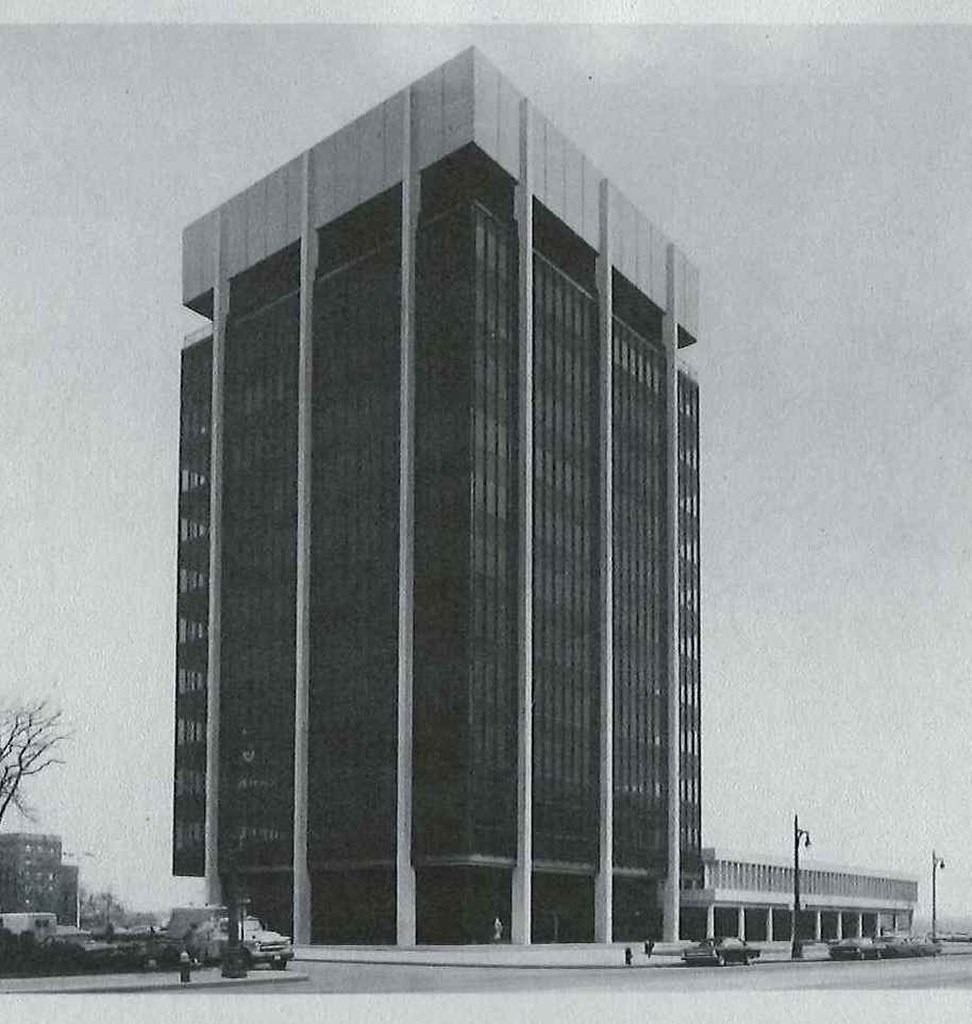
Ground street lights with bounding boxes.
[930,849,945,938]
[790,812,813,961]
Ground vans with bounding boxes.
[1,912,57,947]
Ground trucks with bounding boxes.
[169,904,295,973]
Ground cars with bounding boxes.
[904,935,941,957]
[50,919,177,969]
[868,936,914,959]
[829,936,885,961]
[682,935,760,965]
